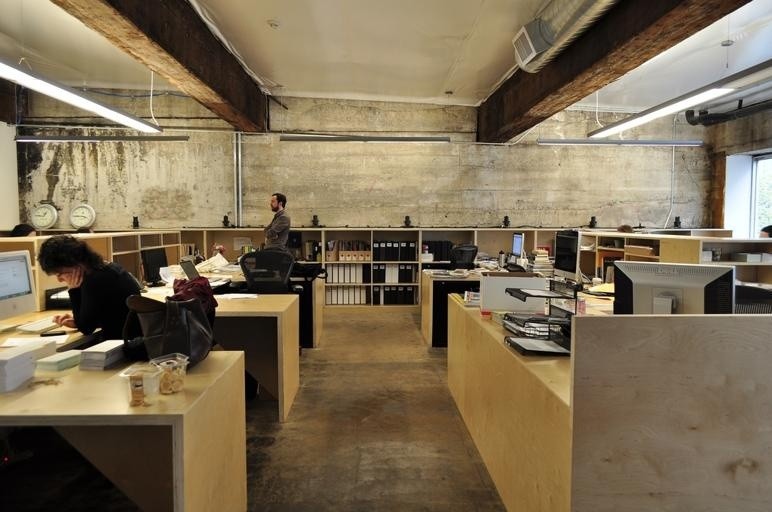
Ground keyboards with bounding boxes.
[16,314,63,334]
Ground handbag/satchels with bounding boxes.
[279,263,328,283]
[122,294,214,370]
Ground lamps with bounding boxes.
[586,13,772,140]
[1,0,163,135]
[278,86,454,144]
[13,56,190,142]
[537,123,704,147]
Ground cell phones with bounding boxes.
[40,330,66,337]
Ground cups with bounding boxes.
[555,276,567,282]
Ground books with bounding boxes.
[422,253,434,262]
[431,268,470,278]
[0,339,127,393]
[527,249,554,272]
[463,290,480,307]
[702,250,772,262]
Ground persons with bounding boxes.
[263,192,293,249]
[36,233,148,362]
[618,224,635,233]
[75,227,96,233]
[759,225,772,238]
[9,223,37,237]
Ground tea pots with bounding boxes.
[592,278,602,287]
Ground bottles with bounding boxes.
[578,298,587,315]
[499,250,504,268]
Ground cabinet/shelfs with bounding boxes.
[32,224,771,306]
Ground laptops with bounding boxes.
[180,260,222,283]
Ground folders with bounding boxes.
[373,286,380,304]
[373,240,416,261]
[373,264,385,283]
[384,286,414,304]
[399,264,412,283]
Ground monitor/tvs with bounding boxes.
[554,230,583,285]
[0,250,38,331]
[140,247,168,287]
[614,260,735,315]
[513,232,526,259]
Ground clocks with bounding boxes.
[69,204,96,229]
[31,204,57,230]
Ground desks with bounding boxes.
[447,293,772,512]
[1,294,300,423]
[1,350,247,512]
[142,272,323,348]
[423,267,551,346]
